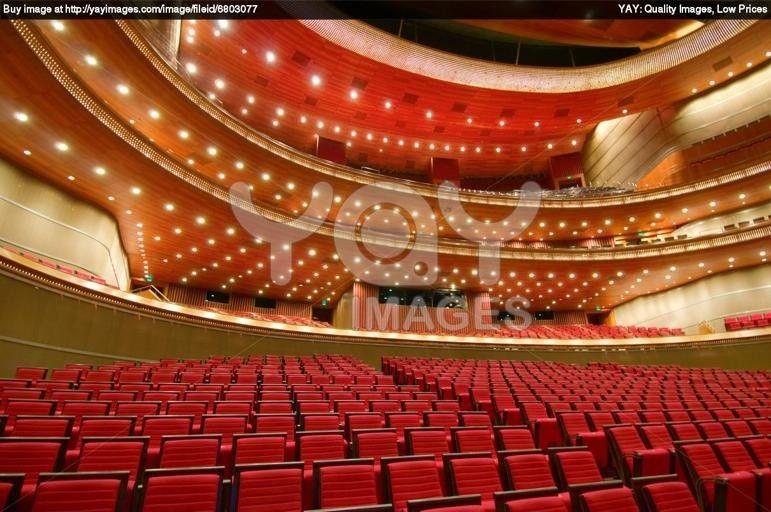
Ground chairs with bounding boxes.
[239,312,771,337]
[0,355,771,512]
